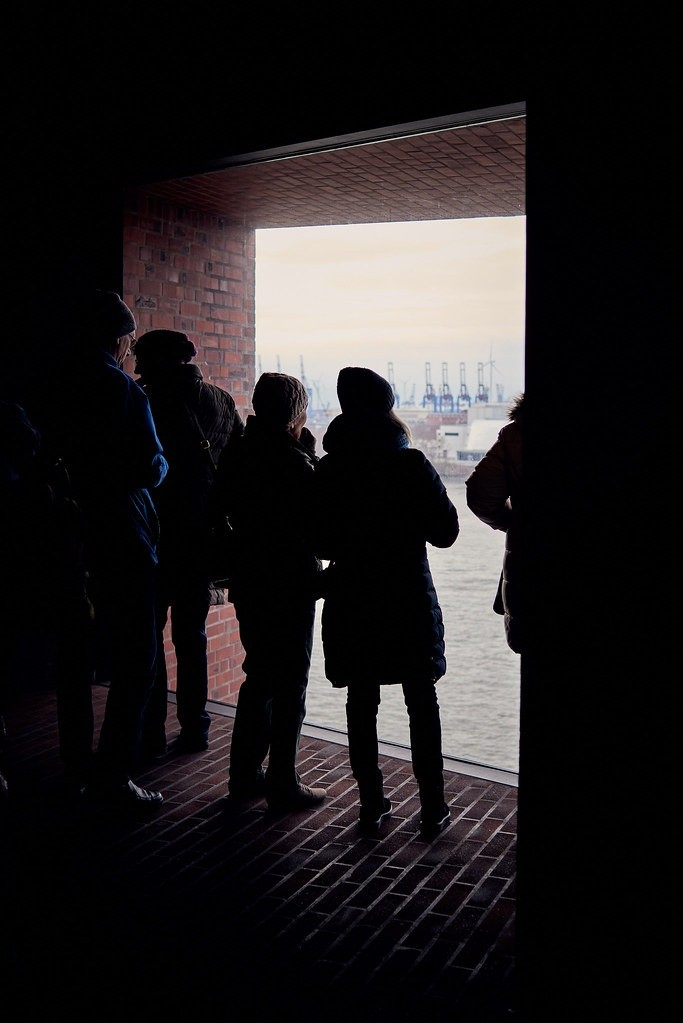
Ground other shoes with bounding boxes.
[359,798,391,823]
[420,803,451,824]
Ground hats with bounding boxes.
[338,369,394,413]
[87,289,136,341]
[253,373,307,424]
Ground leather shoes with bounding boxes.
[84,778,164,812]
[267,786,325,812]
[228,776,267,798]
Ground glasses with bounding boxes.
[128,333,138,347]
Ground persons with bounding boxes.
[303,367,453,832]
[224,372,332,811]
[465,393,524,658]
[0,281,241,815]
